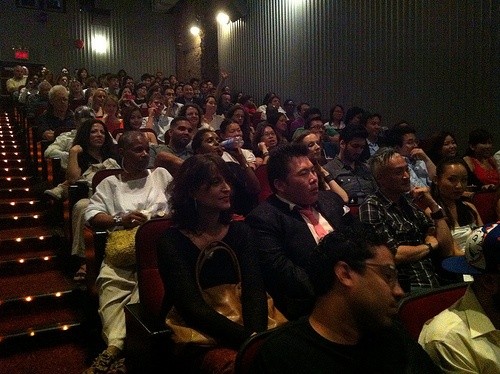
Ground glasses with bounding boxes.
[360,261,397,281]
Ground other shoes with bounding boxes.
[82,349,125,374]
[73,263,87,281]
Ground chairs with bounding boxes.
[14,90,500,374]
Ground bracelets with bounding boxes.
[430,209,444,219]
[424,243,433,250]
[114,215,122,224]
[324,175,334,183]
[263,152,269,157]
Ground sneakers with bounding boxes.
[48,183,65,199]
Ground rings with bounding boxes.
[132,219,135,222]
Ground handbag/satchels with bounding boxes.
[166,240,289,345]
[106,225,138,268]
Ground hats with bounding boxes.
[442,221,500,277]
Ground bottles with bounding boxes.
[218,135,244,153]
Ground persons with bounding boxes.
[7,65,500,374]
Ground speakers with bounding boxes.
[225,0,249,23]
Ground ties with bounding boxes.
[299,206,328,239]
